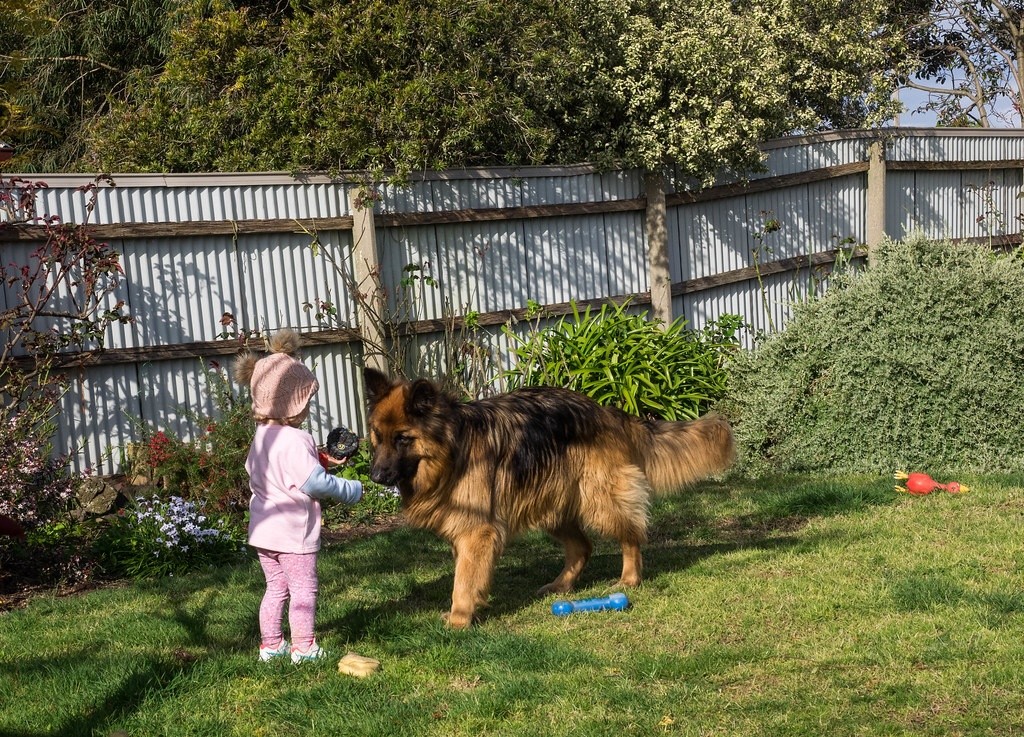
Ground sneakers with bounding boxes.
[291,637,334,665]
[258,633,291,663]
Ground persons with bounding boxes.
[234,328,362,664]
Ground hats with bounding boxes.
[232,327,319,418]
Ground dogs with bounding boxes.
[363,367,735,636]
